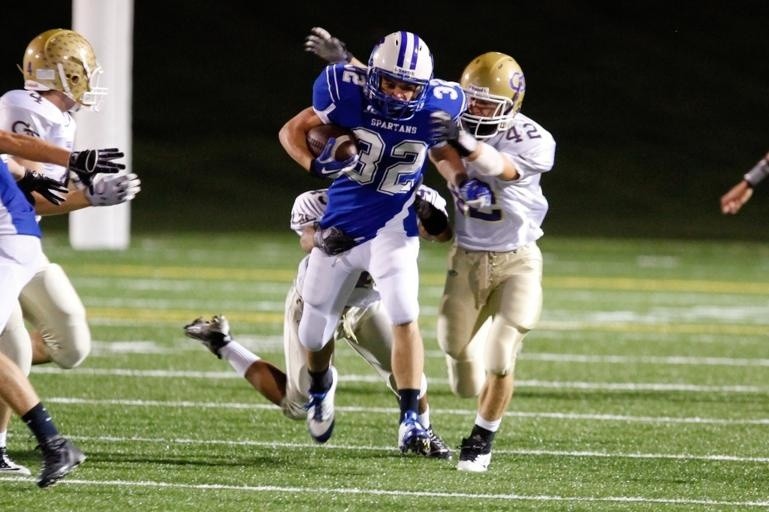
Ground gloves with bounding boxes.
[309,137,359,179]
[313,226,356,255]
[304,27,353,64]
[430,110,477,157]
[16,168,69,207]
[453,177,496,217]
[413,184,447,236]
[85,173,141,206]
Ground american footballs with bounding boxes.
[307,126,358,161]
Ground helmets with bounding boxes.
[23,29,107,113]
[364,31,434,121]
[460,52,526,139]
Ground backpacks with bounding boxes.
[69,148,125,196]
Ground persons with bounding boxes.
[0,26,144,476]
[305,22,557,473]
[0,128,128,489]
[720,148,769,218]
[271,25,496,455]
[182,176,454,459]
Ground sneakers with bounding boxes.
[33,434,88,488]
[425,424,449,459]
[0,446,30,475]
[456,437,491,472]
[398,410,431,456]
[304,365,338,444]
[183,316,231,359]
